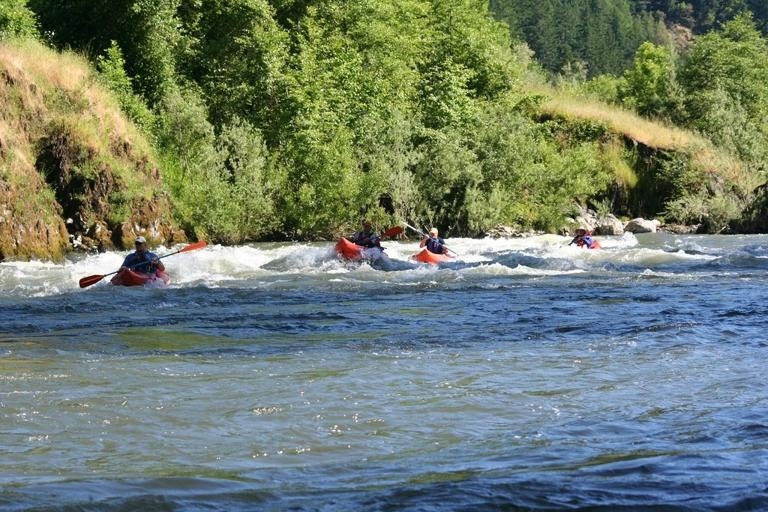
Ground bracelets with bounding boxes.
[158,260,161,264]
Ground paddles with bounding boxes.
[79,240,206,287]
[402,221,458,255]
[369,226,402,240]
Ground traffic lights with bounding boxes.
[576,240,600,249]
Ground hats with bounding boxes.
[575,226,587,232]
[134,236,146,243]
[430,228,438,239]
[363,221,371,228]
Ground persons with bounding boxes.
[119,236,165,278]
[420,228,448,254]
[568,224,593,249]
[352,222,378,248]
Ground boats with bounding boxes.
[334,237,381,260]
[111,266,170,287]
[411,247,449,264]
